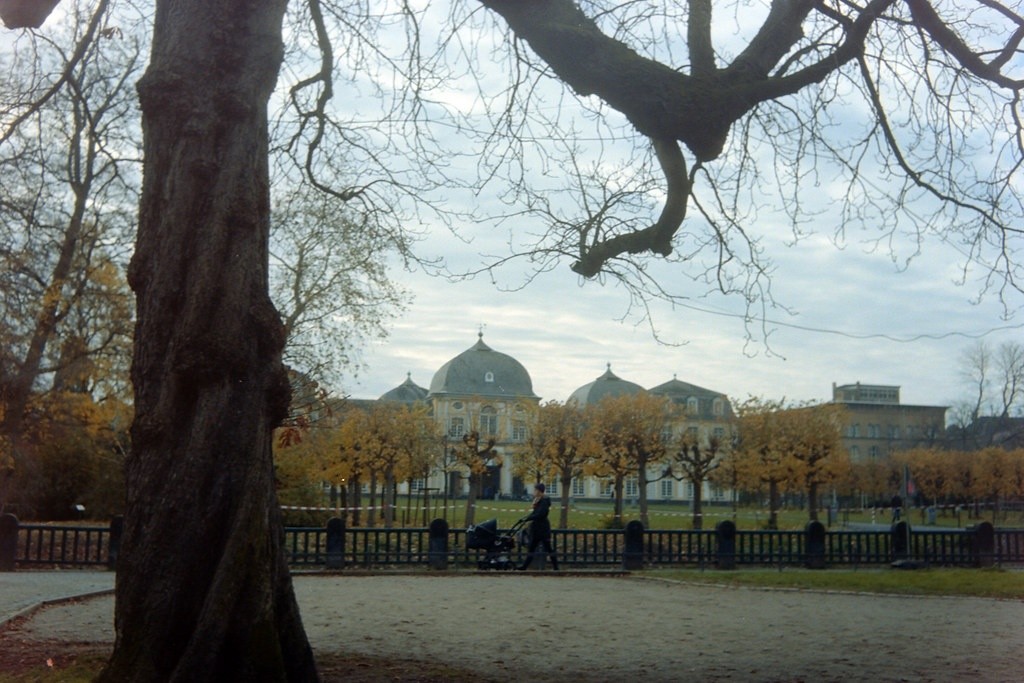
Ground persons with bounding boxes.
[515,483,559,571]
[890,490,903,523]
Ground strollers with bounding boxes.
[466,516,525,571]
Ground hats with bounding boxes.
[535,484,545,493]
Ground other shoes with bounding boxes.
[554,565,559,570]
[516,567,526,570]
